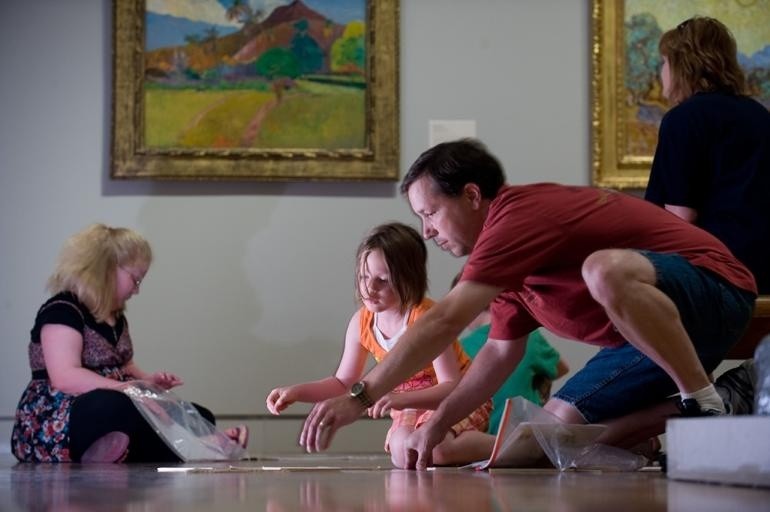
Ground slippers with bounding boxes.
[80,432,129,463]
[218,425,249,455]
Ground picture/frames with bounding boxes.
[588,0,770,192]
[111,0,403,183]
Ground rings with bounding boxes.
[317,420,326,429]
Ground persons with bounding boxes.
[445,267,565,434]
[299,140,759,471]
[10,225,250,464]
[643,15,767,296]
[269,224,497,467]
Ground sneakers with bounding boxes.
[647,358,756,470]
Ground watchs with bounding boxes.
[348,378,374,409]
